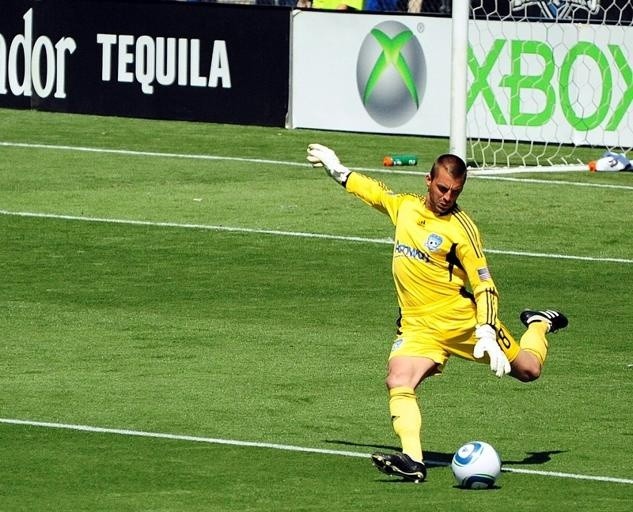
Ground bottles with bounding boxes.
[383,154,418,166]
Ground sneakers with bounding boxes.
[520,310,569,333]
[371,452,427,484]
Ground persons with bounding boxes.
[362,0,452,12]
[296,0,363,13]
[306,144,569,485]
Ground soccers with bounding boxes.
[450,441,500,489]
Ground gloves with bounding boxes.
[306,142,352,188]
[473,325,515,378]
